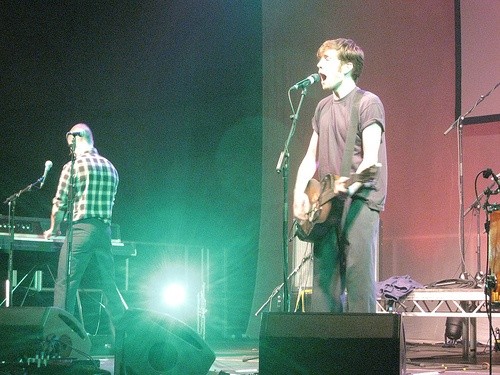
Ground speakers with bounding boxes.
[114,308,216,375]
[258,311,407,375]
[0,306,92,363]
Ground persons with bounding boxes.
[43,123,130,353]
[292,38,388,313]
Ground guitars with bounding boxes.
[291,165,381,243]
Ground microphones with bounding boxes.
[290,73,320,90]
[67,130,84,136]
[40,160,53,188]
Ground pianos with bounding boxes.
[0,215,138,259]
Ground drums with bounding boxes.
[487,201,500,309]
